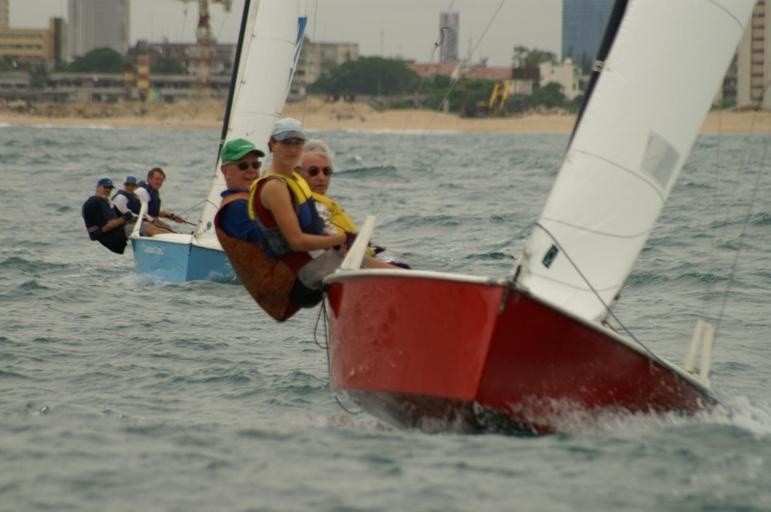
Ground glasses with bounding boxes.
[309,167,333,176]
[239,162,262,170]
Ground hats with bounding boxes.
[124,176,137,185]
[100,179,115,188]
[272,117,305,141]
[221,139,265,163]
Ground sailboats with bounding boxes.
[124,1,328,292]
[318,0,763,442]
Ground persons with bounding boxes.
[294,139,386,257]
[213,139,412,322]
[82,177,169,249]
[137,167,183,228]
[111,174,174,236]
[247,116,404,290]
